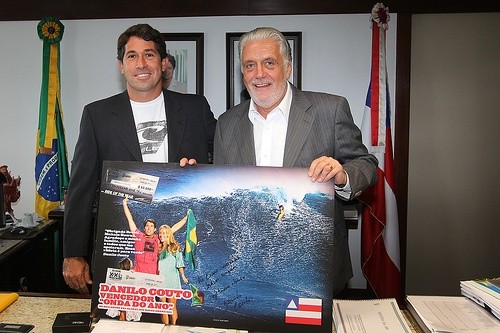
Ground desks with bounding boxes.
[0,203,500,333]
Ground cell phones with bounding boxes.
[0,323,34,333]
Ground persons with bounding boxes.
[117,197,190,325]
[62,23,216,296]
[213,27,379,298]
[162,52,176,89]
[0,165,22,221]
[358,2,403,310]
[35,16,69,221]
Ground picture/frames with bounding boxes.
[160,32,205,96]
[225,31,302,112]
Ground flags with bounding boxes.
[189,283,204,308]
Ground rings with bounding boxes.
[326,163,333,170]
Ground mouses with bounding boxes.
[11,227,28,234]
